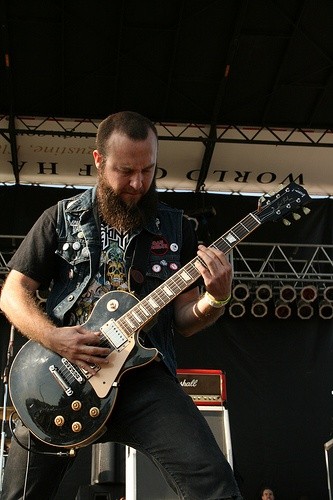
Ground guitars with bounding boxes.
[8,180,314,449]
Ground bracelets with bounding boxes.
[205,291,231,308]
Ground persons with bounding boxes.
[262,488,275,500]
[0,111,244,500]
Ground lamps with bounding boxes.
[230,283,333,320]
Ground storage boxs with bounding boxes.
[125,406,235,500]
